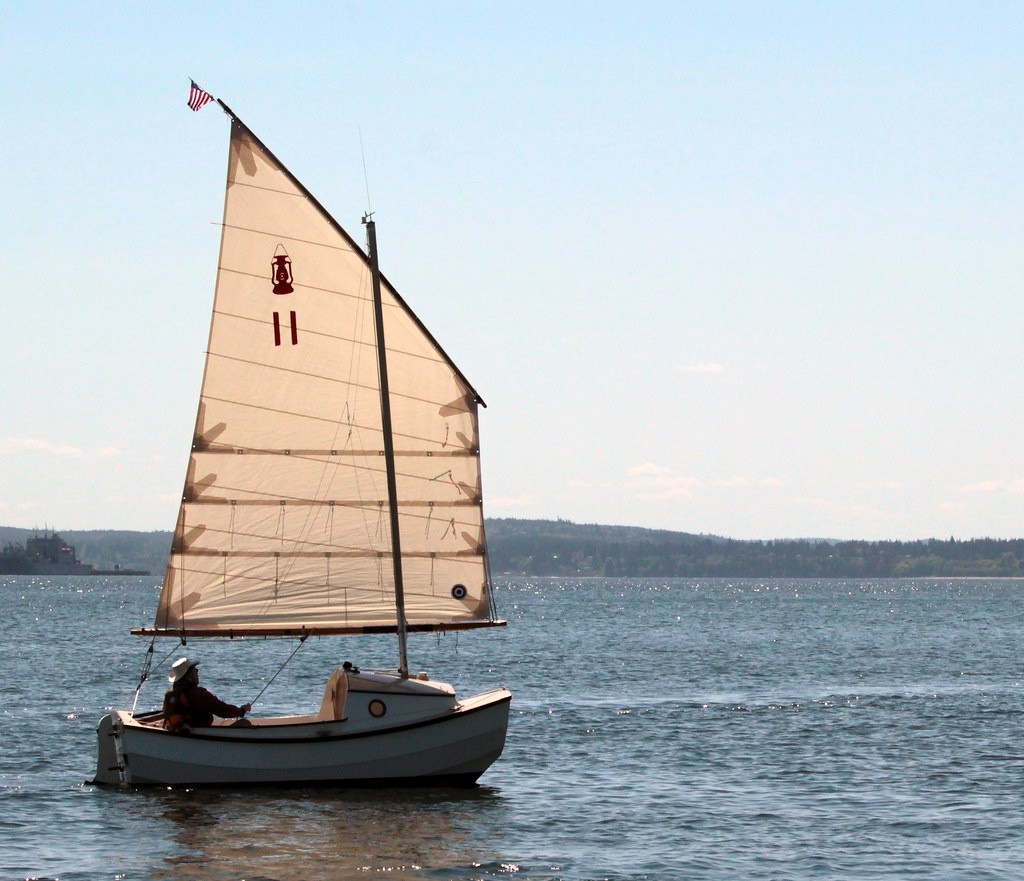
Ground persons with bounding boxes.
[163,657,251,727]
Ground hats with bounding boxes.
[167,656,200,683]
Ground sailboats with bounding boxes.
[84,97,513,789]
